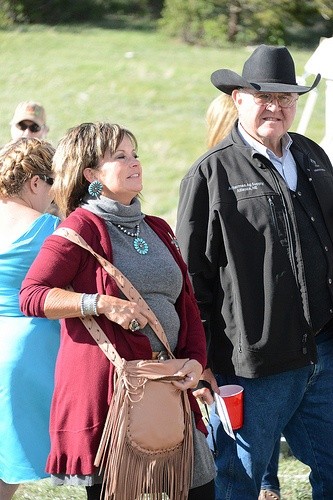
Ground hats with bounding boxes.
[9,101,46,128]
[210,44,321,96]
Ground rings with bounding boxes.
[130,320,140,332]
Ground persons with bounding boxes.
[206,93,281,500]
[0,136,62,500]
[20,124,218,500]
[176,47,333,500]
[11,102,61,218]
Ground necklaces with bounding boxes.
[116,224,149,254]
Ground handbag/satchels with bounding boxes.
[94,357,195,500]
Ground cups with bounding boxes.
[214,385,244,430]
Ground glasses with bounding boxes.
[14,120,41,132]
[238,88,299,108]
